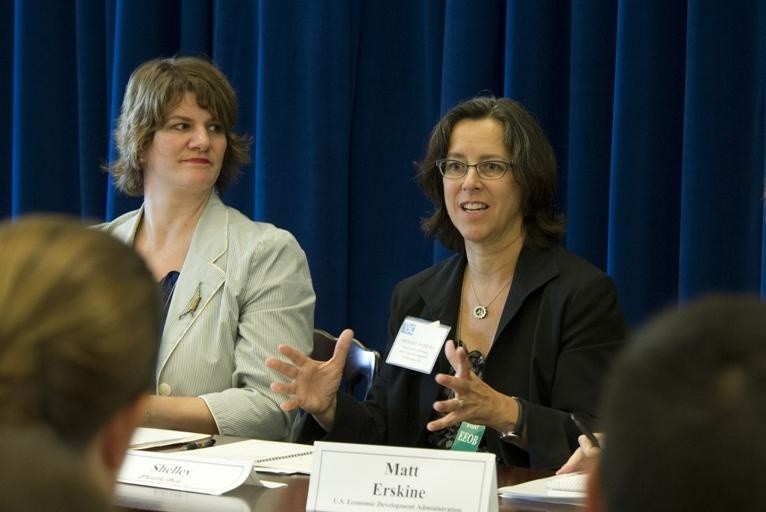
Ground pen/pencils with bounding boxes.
[570,413,601,448]
[180,439,216,450]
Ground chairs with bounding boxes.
[299,328,381,445]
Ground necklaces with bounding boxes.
[468,271,514,320]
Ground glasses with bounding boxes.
[437,159,513,181]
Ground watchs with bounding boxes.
[499,398,524,440]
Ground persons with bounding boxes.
[265,96,627,472]
[586,297,766,512]
[0,215,160,512]
[555,432,606,477]
[83,57,316,441]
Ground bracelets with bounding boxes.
[143,395,153,428]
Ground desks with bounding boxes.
[117,433,587,512]
